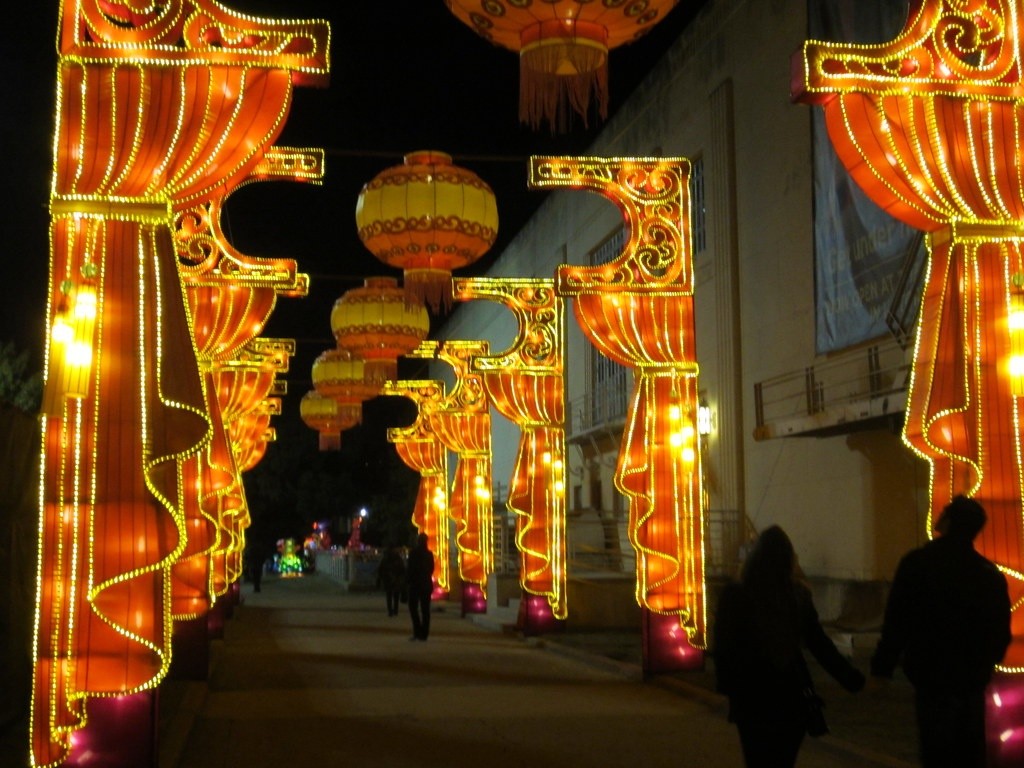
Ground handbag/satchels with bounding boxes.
[802,687,829,737]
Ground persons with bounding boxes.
[702,524,869,768]
[375,542,407,617]
[870,492,1013,768]
[399,532,436,642]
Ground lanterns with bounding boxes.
[355,150,499,316]
[330,276,430,390]
[299,390,363,452]
[262,506,371,580]
[445,0,681,134]
[310,342,387,426]
